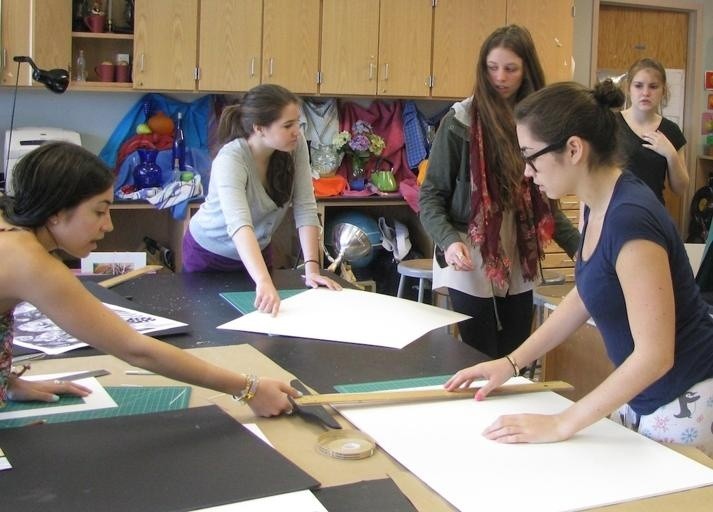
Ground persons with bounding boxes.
[181,85,342,317]
[445,80,712,447]
[417,25,581,360]
[0,141,304,419]
[614,56,690,207]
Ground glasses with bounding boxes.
[519,144,558,173]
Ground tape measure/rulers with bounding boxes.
[98,264,163,290]
[51,369,111,382]
[288,380,575,429]
[332,374,459,395]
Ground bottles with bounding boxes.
[93,61,113,83]
[73,48,87,83]
[171,111,187,172]
[170,156,180,190]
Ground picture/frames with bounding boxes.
[79,251,146,275]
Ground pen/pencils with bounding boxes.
[18,419,47,427]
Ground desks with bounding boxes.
[0,270,711,512]
[108,201,204,274]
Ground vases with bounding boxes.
[350,160,366,191]
[133,147,162,191]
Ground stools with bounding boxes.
[395,258,454,334]
[524,267,572,381]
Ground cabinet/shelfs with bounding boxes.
[29,1,196,95]
[318,0,431,102]
[430,0,574,103]
[0,0,31,88]
[196,0,319,96]
[532,190,580,283]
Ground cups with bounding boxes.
[82,13,106,33]
[113,60,133,83]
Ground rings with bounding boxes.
[451,262,454,266]
[284,410,292,414]
[456,251,464,259]
[54,380,64,386]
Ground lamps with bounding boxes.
[0,55,71,194]
[316,219,375,291]
[597,67,629,114]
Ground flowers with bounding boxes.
[333,118,385,160]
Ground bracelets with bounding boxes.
[505,354,519,377]
[303,260,318,265]
[232,373,258,404]
